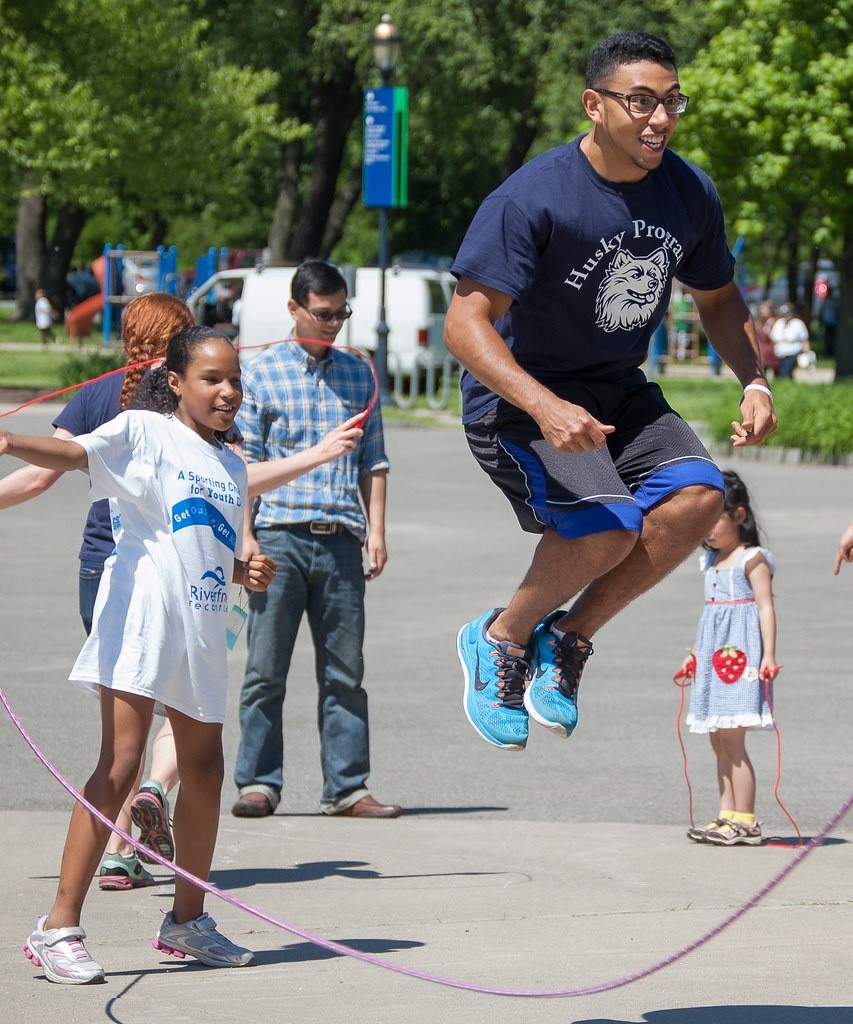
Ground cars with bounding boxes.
[741,257,842,334]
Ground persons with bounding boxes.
[0,325,277,985]
[686,470,780,847]
[34,288,60,346]
[757,300,811,382]
[232,260,402,820]
[0,292,367,890]
[443,30,777,750]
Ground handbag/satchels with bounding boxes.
[797,351,816,367]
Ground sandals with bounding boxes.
[701,821,765,845]
[687,817,730,841]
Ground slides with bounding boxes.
[65,292,102,338]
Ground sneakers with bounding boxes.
[456,608,533,751]
[152,907,255,968]
[23,916,105,985]
[524,610,594,738]
[98,851,154,889]
[130,779,174,864]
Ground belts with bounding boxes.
[276,520,347,535]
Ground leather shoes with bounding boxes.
[323,795,401,818]
[232,791,273,816]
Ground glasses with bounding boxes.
[297,302,353,322]
[592,89,689,114]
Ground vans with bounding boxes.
[179,263,460,393]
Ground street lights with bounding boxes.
[367,7,406,409]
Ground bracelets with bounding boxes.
[743,384,774,404]
